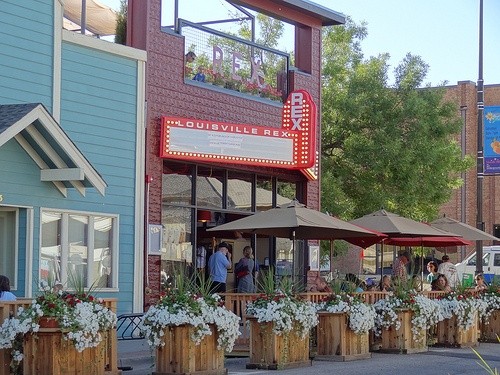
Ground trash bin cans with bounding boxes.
[462,272,473,287]
[483,273,495,284]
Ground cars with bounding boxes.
[378,256,443,275]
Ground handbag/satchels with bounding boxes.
[235,265,250,278]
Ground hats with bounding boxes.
[400,252,411,258]
[219,242,230,249]
[442,255,450,260]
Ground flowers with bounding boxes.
[372,295,442,331]
[0,287,118,371]
[246,290,319,338]
[143,288,245,353]
[315,294,378,335]
[477,291,500,315]
[433,294,481,331]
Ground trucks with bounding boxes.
[454,245,500,284]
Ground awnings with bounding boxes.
[61,0,124,39]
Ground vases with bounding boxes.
[315,309,373,362]
[479,303,500,343]
[245,312,316,372]
[152,322,230,375]
[21,322,122,375]
[429,296,481,348]
[378,304,431,356]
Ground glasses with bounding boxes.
[475,278,482,280]
[405,256,409,261]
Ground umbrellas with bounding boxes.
[427,213,500,242]
[348,207,463,237]
[342,230,472,250]
[205,198,389,296]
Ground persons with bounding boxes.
[234,246,255,326]
[0,275,17,303]
[309,275,393,304]
[192,65,206,83]
[391,251,411,292]
[411,274,422,292]
[185,51,196,76]
[437,255,462,289]
[207,241,232,300]
[431,279,440,291]
[427,260,441,285]
[474,273,488,291]
[432,274,451,300]
